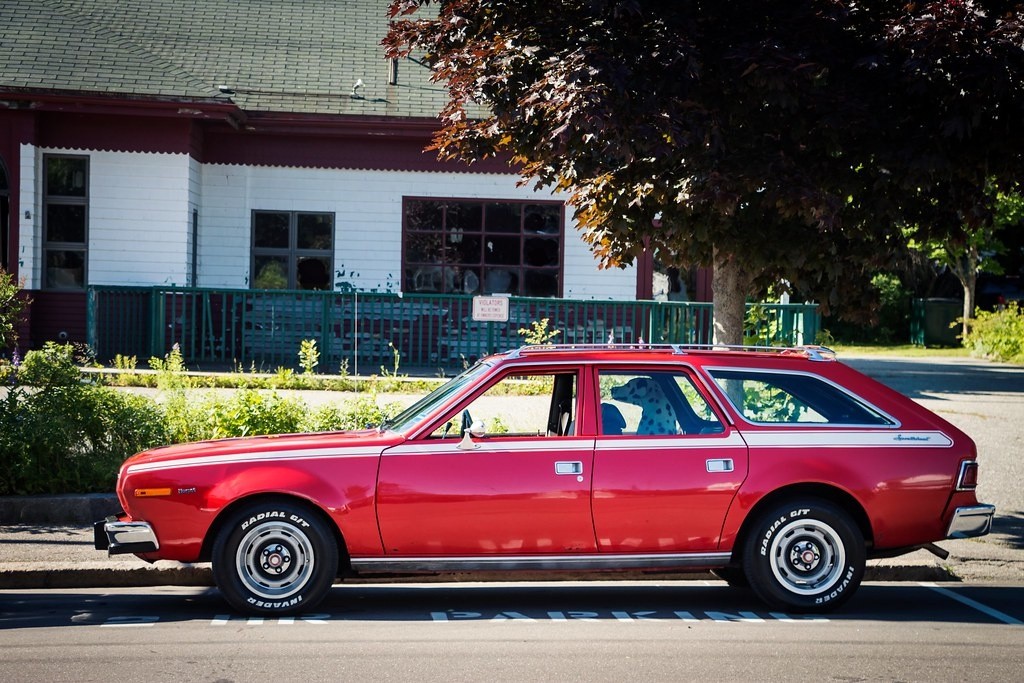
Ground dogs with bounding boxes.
[611,379,677,434]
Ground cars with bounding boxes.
[94,342,996,615]
